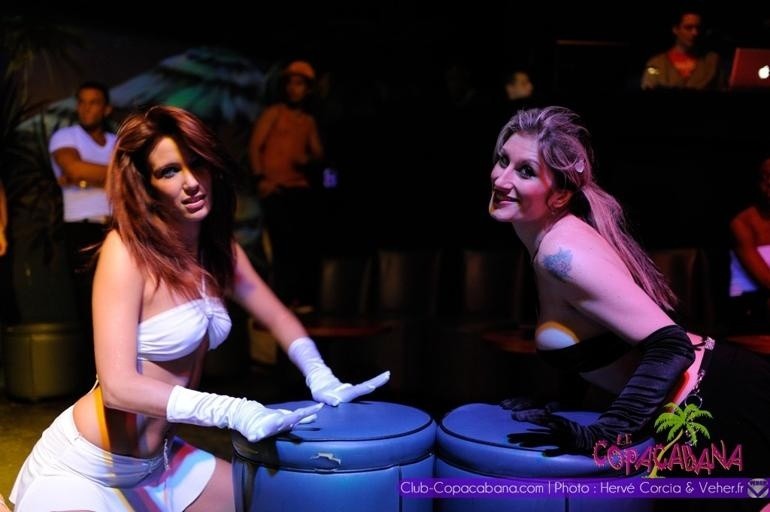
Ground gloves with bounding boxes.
[501,396,565,414]
[288,337,391,405]
[524,324,696,456]
[166,385,324,441]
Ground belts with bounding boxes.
[79,217,114,224]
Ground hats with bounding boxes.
[285,62,315,78]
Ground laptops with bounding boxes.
[696,48,770,91]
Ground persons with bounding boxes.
[486,104,770,511]
[504,69,534,100]
[640,7,726,90]
[247,60,327,199]
[47,83,119,341]
[9,104,393,512]
[729,156,770,335]
